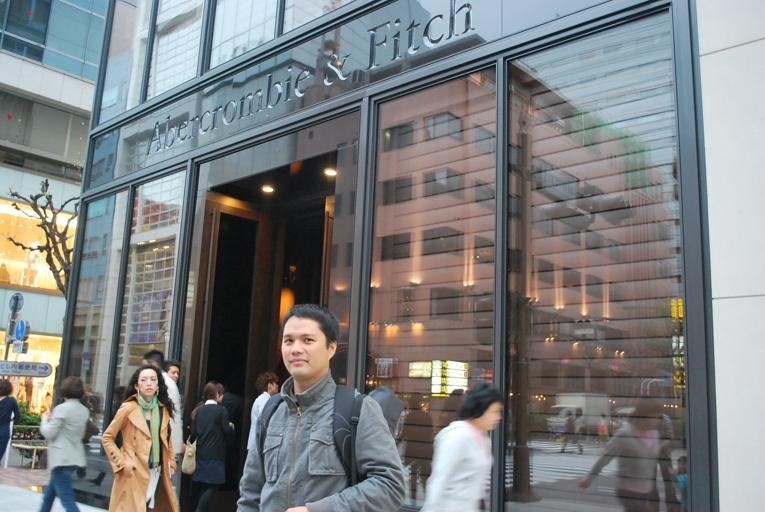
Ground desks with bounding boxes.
[11,443,48,469]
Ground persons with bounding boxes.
[366,383,408,469]
[244,372,280,450]
[101,365,181,511]
[76,388,101,479]
[234,305,408,510]
[85,385,128,487]
[579,398,664,511]
[640,376,683,511]
[0,379,22,460]
[401,388,436,499]
[597,412,609,441]
[38,376,90,512]
[559,408,584,455]
[163,359,180,384]
[438,389,467,431]
[180,381,236,511]
[142,349,181,476]
[669,457,688,511]
[416,381,505,511]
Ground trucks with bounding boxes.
[545,392,611,443]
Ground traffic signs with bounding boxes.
[12,341,24,353]
[0,360,53,378]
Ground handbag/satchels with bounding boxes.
[82,420,100,444]
[181,435,196,475]
